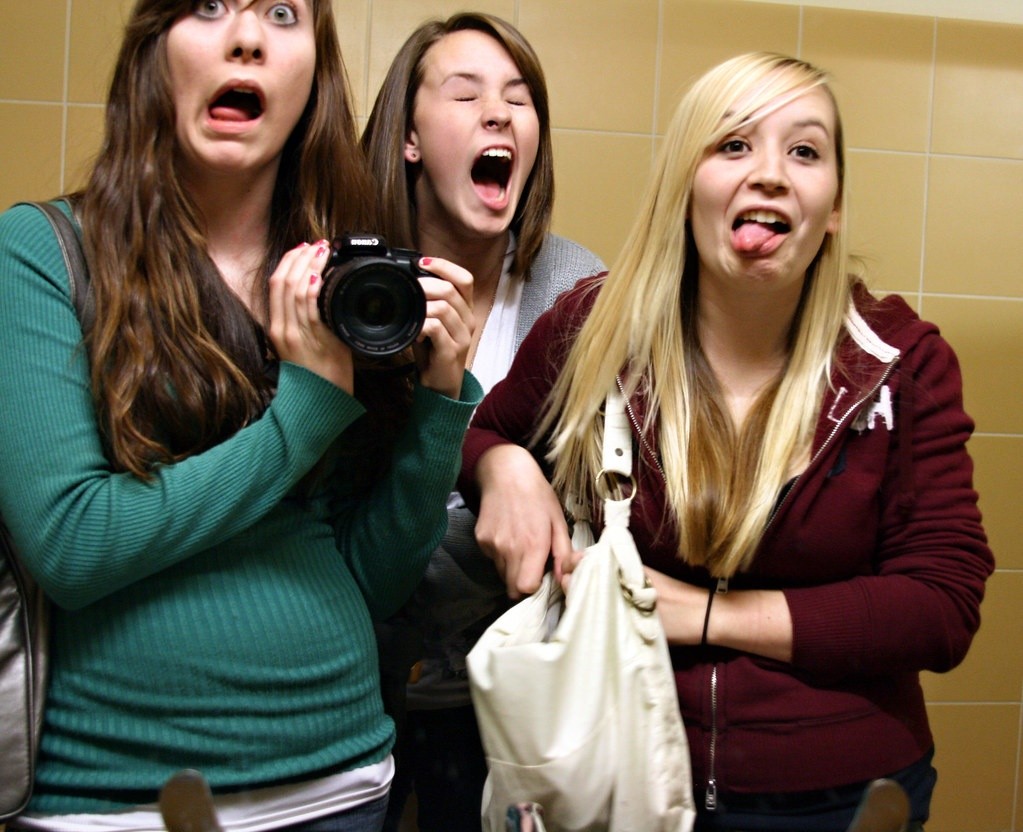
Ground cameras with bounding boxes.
[316,233,437,357]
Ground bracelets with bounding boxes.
[702,588,716,644]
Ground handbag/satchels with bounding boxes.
[462,377,696,832]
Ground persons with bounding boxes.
[0,0,484,832]
[357,10,605,832]
[457,52,997,832]
